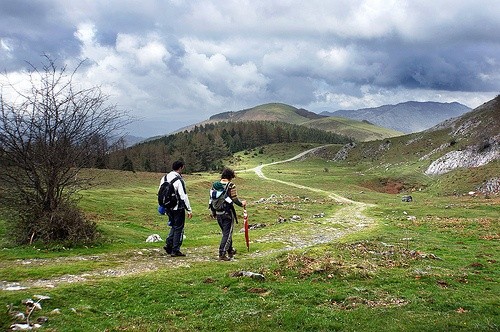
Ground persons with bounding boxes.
[208,168,247,261]
[156,160,193,257]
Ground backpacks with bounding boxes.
[158,173,181,209]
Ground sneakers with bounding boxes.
[165,246,173,254]
[218,255,230,261]
[171,250,186,257]
[228,248,236,257]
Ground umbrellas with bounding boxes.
[243,205,249,252]
